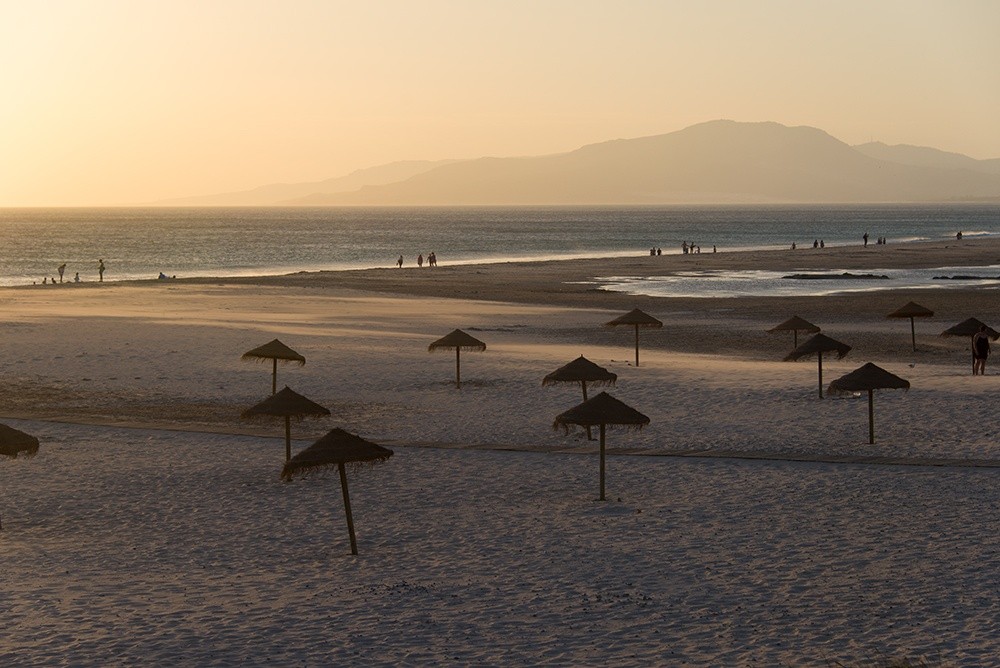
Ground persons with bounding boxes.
[971,324,992,377]
[33,229,964,286]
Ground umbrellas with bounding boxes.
[604,306,664,369]
[240,336,309,398]
[279,425,396,555]
[939,314,1000,374]
[0,422,42,462]
[826,361,910,445]
[540,353,621,441]
[242,382,329,462]
[766,313,822,351]
[428,327,488,389]
[782,331,850,400]
[887,300,936,352]
[551,389,653,502]
